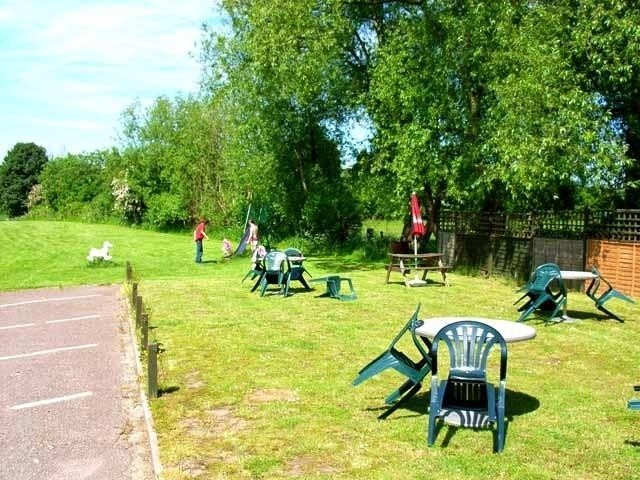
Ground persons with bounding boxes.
[222,239,231,255]
[193,219,211,263]
[245,219,257,254]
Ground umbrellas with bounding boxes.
[407,189,424,268]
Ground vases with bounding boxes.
[392,240,409,254]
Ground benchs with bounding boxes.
[385,263,454,288]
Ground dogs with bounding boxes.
[87,241,114,262]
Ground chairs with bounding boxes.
[309,275,358,302]
[241,245,313,299]
[351,302,507,454]
[512,263,567,326]
[587,265,635,323]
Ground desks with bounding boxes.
[415,316,537,368]
[386,252,448,287]
[530,268,599,324]
